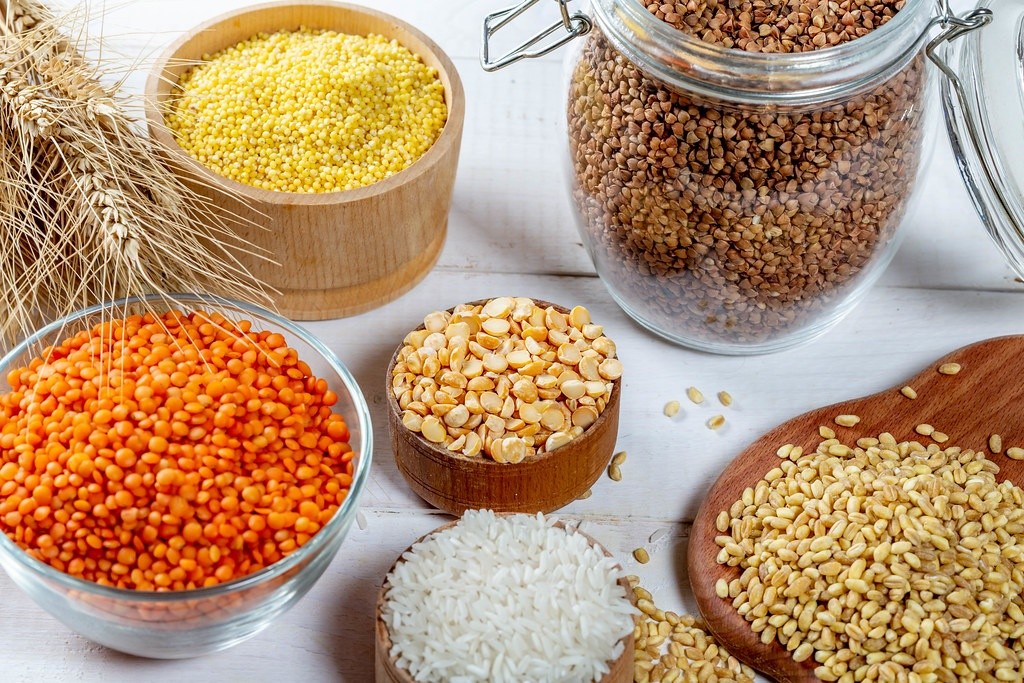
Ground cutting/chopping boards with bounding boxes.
[687,334,1024,683]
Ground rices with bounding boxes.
[379,508,643,683]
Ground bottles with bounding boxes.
[482,0,941,358]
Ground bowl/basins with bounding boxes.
[384,295,622,518]
[373,512,634,683]
[143,1,466,320]
[0,292,374,660]
